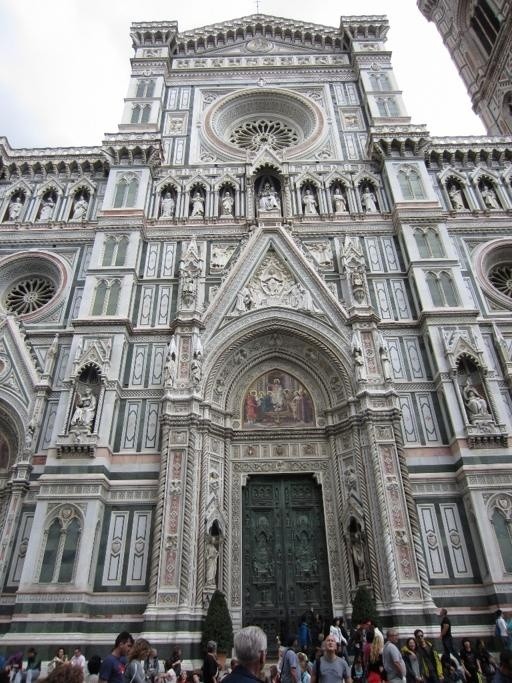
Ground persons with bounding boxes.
[191,191,205,217]
[333,188,347,212]
[351,532,368,582]
[71,388,96,433]
[245,375,311,428]
[303,189,317,214]
[257,183,281,212]
[450,183,465,211]
[206,535,220,588]
[39,197,54,222]
[466,391,488,416]
[160,192,175,219]
[0,607,512,683]
[481,184,500,208]
[8,197,22,221]
[73,195,87,221]
[361,186,379,213]
[221,191,234,215]
